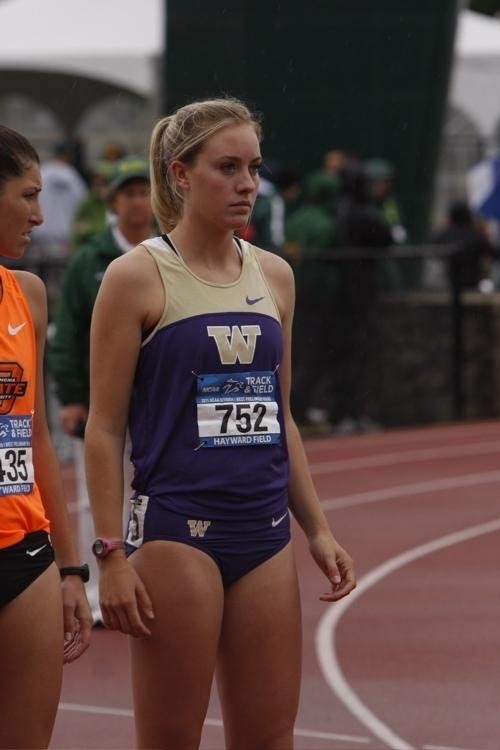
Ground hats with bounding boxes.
[109,152,154,197]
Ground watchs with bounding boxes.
[90,536,128,556]
[57,564,89,583]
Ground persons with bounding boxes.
[0,124,91,750]
[45,156,173,629]
[84,97,356,748]
[36,130,499,423]
